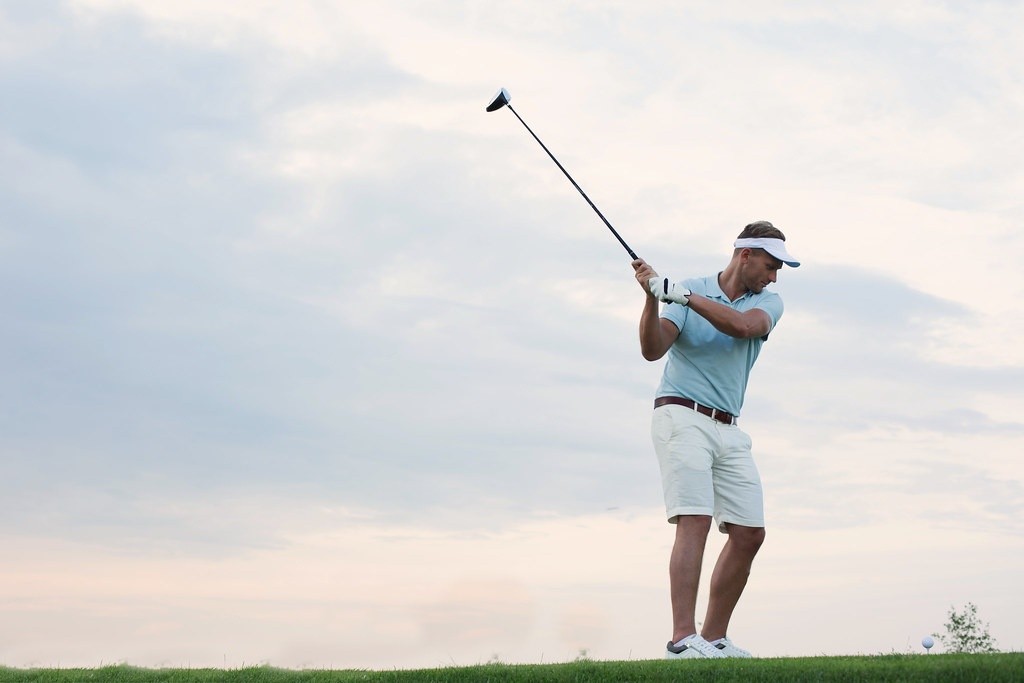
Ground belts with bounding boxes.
[654,396,737,426]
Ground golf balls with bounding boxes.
[921,636,934,649]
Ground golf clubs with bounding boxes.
[485,87,673,305]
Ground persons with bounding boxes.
[631,222,801,659]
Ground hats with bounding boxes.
[733,237,800,267]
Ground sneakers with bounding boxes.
[666,634,727,659]
[709,637,753,657]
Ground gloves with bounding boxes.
[649,277,692,307]
